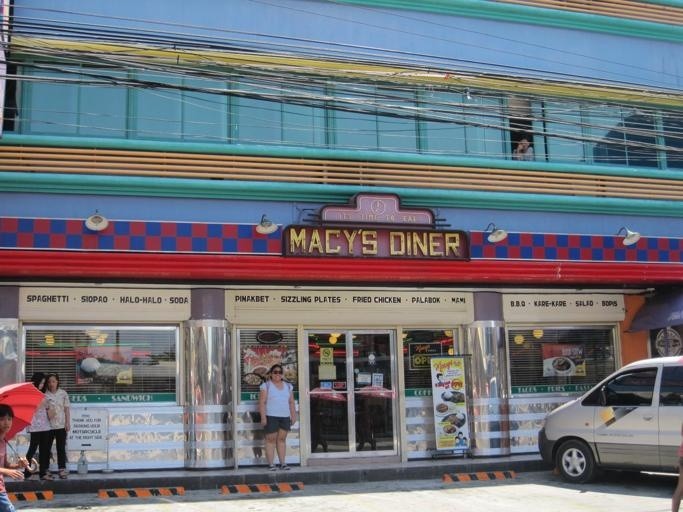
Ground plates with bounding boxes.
[436,390,466,436]
[543,357,575,376]
[243,366,271,387]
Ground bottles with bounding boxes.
[77,450,88,476]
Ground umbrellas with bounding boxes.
[311,388,347,403]
[358,384,393,399]
[0,381,47,473]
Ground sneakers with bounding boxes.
[278,463,290,470]
[39,471,55,480]
[57,470,66,478]
[267,464,276,471]
[23,470,33,479]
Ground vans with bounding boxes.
[538,356,683,484]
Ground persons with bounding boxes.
[354,397,378,451]
[313,398,329,452]
[436,373,444,386]
[42,372,71,480]
[23,371,54,482]
[0,403,30,511]
[455,431,467,446]
[511,137,534,160]
[258,364,296,471]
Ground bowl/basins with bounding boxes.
[551,357,572,374]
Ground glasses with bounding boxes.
[272,370,282,374]
